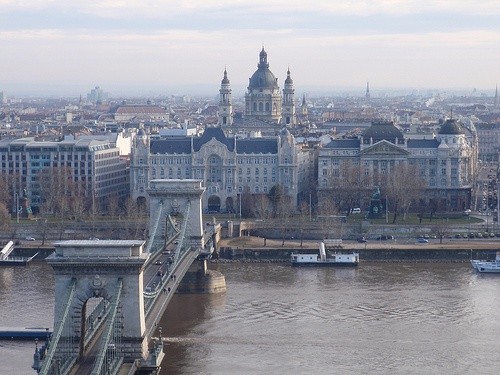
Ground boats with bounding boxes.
[470,251,500,273]
[290,253,360,267]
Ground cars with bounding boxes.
[479,168,499,222]
[144,238,179,291]
[357,232,499,243]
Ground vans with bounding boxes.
[345,208,361,214]
